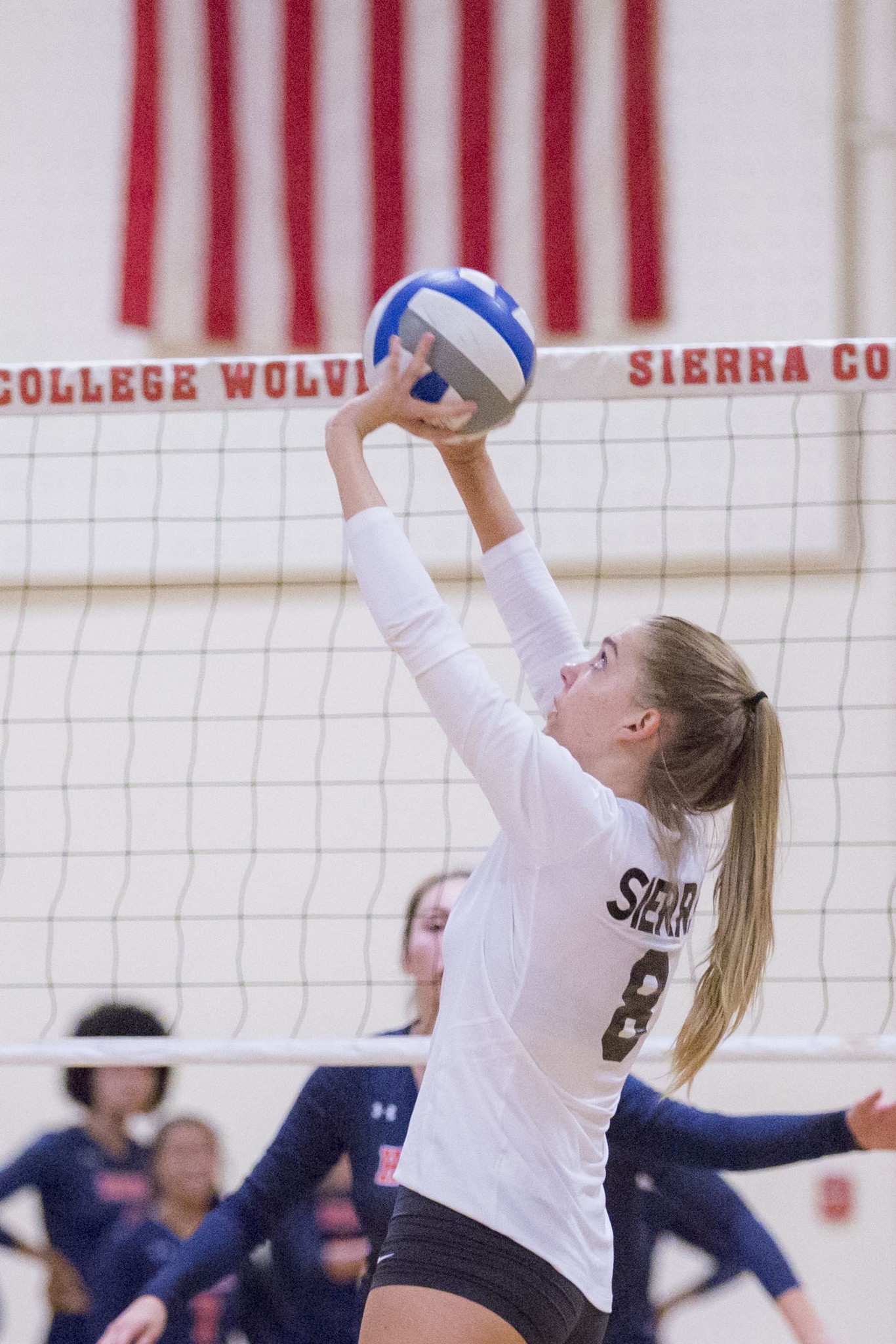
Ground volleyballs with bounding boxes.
[362,265,539,435]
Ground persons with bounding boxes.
[0,871,896,1344]
[323,331,789,1344]
